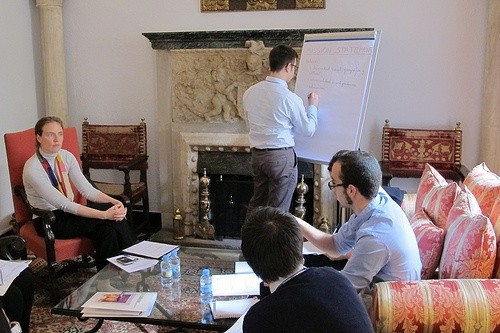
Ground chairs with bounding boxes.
[378,119,469,186]
[79,116,151,241]
[4,126,133,306]
[0,234,29,333]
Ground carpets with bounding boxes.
[30,258,234,333]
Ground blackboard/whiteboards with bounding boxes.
[291,30,376,164]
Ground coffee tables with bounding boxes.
[50,247,264,333]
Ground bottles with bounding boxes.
[173,209,184,239]
[200,269,212,303]
[320,218,328,234]
[161,255,173,287]
[169,250,181,282]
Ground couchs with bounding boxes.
[372,163,500,333]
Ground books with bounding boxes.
[209,298,260,318]
[106,241,181,274]
[212,273,261,297]
[81,291,157,317]
[235,262,256,273]
[0,259,32,296]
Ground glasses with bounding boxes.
[286,64,298,71]
[328,180,349,191]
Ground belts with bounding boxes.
[252,147,293,152]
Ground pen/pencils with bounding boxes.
[119,207,128,220]
[307,95,311,97]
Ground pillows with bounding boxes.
[437,185,495,278]
[409,208,445,280]
[415,163,456,228]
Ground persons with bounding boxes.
[22,116,132,271]
[293,150,422,294]
[224,205,374,333]
[0,270,37,333]
[243,45,319,220]
